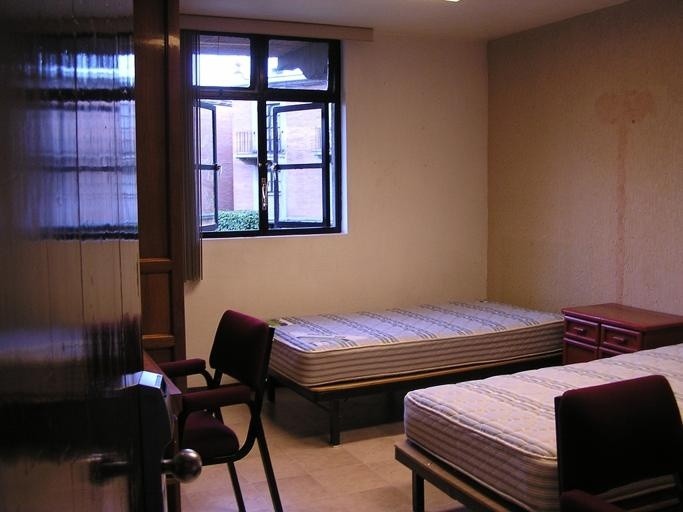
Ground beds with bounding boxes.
[393,339,683,511]
[263,296,565,444]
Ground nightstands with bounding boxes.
[561,302,683,364]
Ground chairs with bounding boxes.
[541,374,683,512]
[159,310,286,512]
